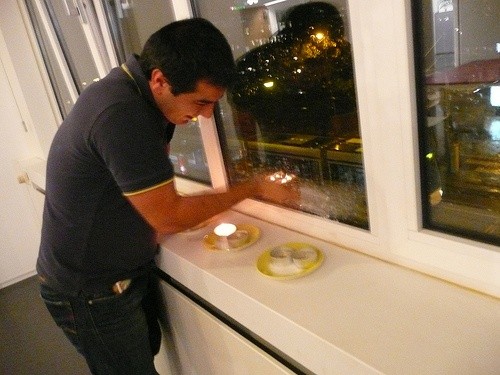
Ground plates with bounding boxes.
[203,224,259,253]
[256,242,323,279]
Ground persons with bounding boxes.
[228,2,367,187]
[35,16,334,375]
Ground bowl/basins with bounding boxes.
[294,248,317,268]
[269,248,294,265]
[227,230,248,247]
